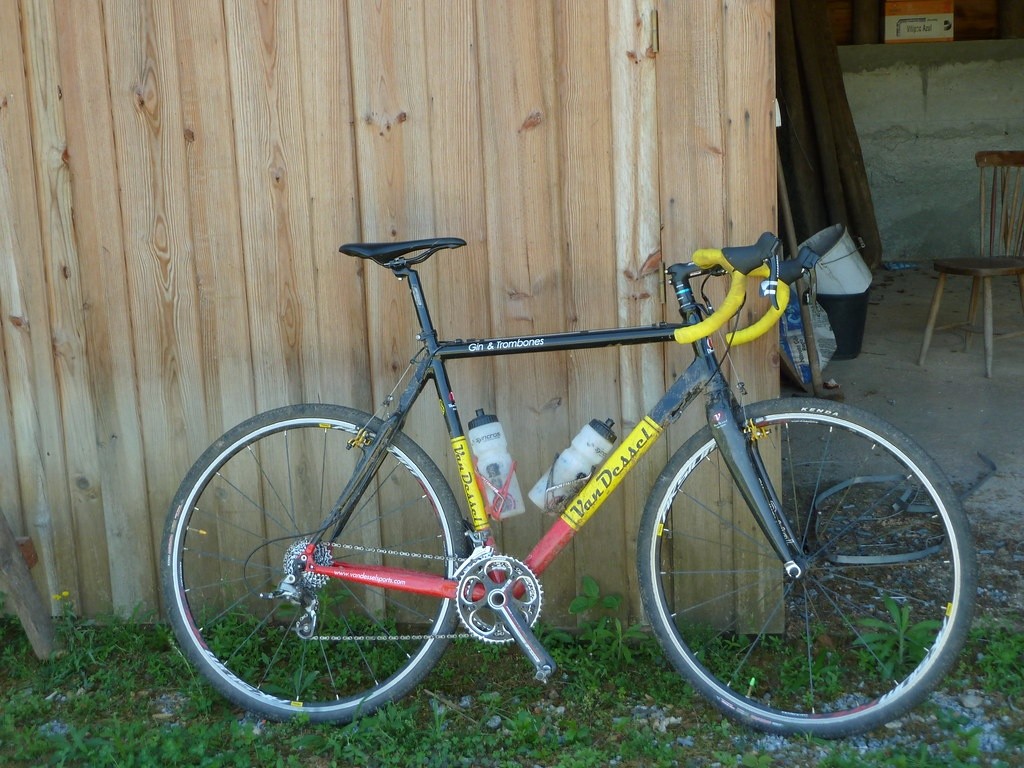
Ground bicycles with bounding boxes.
[152,230,982,740]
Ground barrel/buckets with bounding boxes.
[786,221,874,298]
[807,290,873,364]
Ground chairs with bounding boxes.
[918,150,1024,377]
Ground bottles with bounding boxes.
[527,415,618,520]
[467,407,527,523]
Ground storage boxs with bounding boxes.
[880,0,953,44]
[954,0,999,40]
